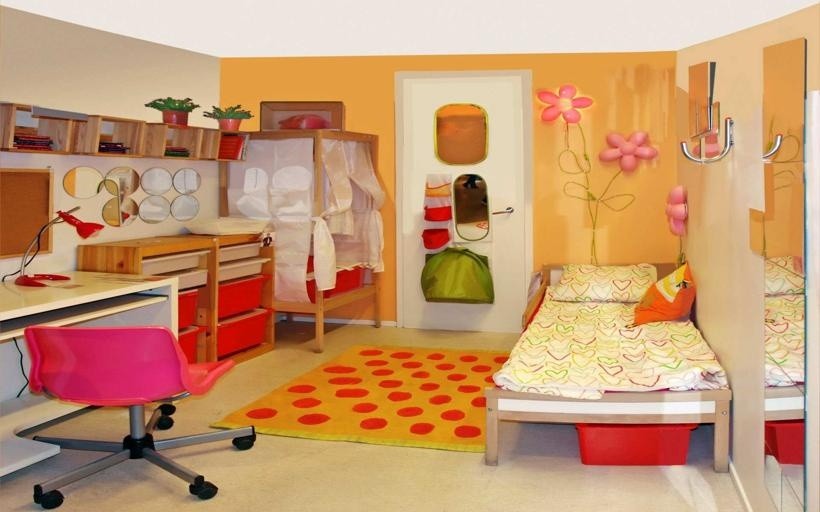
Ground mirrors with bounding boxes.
[64,166,202,227]
[750,38,806,512]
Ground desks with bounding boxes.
[0,271,179,477]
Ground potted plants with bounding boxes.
[145,96,201,125]
[203,105,256,130]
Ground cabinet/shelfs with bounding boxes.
[77,234,275,363]
[0,103,248,162]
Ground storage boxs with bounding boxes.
[174,278,274,363]
[765,420,805,465]
[575,423,699,465]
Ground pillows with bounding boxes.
[551,264,657,303]
[634,263,696,325]
[764,255,805,296]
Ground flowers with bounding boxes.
[537,85,658,268]
[665,185,688,267]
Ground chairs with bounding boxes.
[24,326,256,509]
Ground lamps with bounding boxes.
[15,206,105,287]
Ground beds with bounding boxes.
[484,263,733,472]
[764,257,805,421]
[219,131,381,352]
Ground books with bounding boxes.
[165,151,191,156]
[13,124,56,153]
[166,145,187,151]
[99,140,131,154]
[219,134,250,160]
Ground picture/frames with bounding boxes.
[260,101,343,130]
[0,168,54,260]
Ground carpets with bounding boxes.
[209,346,510,453]
